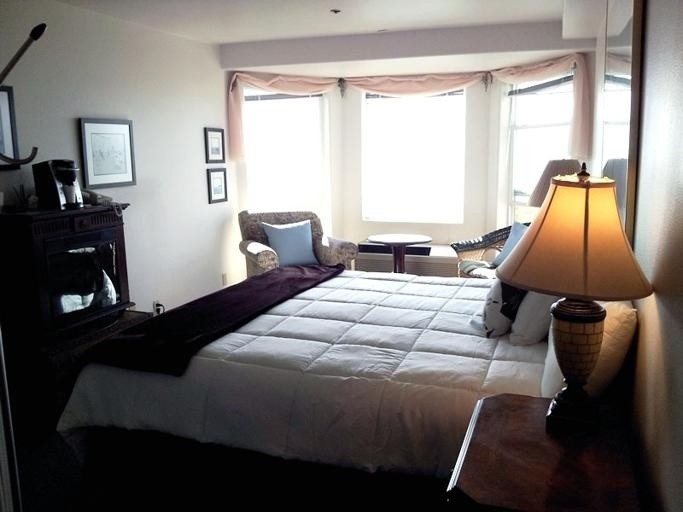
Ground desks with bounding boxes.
[368,234,432,274]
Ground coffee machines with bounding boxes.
[30,158,85,211]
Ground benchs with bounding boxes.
[359,245,458,277]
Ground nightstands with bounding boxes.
[446,393,663,511]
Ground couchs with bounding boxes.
[449,223,531,279]
[238,211,358,279]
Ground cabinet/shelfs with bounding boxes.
[0,203,153,384]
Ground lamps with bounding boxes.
[496,162,654,449]
[527,160,581,207]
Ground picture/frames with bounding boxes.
[205,127,224,163]
[207,168,227,204]
[79,117,136,188]
[0,86,20,172]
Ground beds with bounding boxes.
[46,265,638,511]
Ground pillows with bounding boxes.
[540,320,564,398]
[509,291,565,345]
[484,278,528,339]
[491,222,529,267]
[585,301,637,399]
[262,220,319,268]
